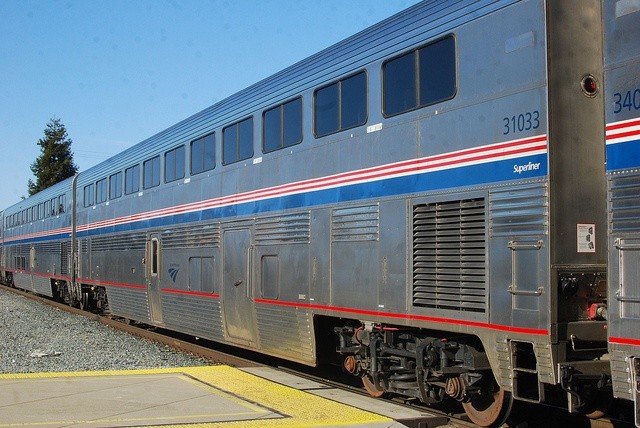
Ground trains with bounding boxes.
[1,1,640,427]
